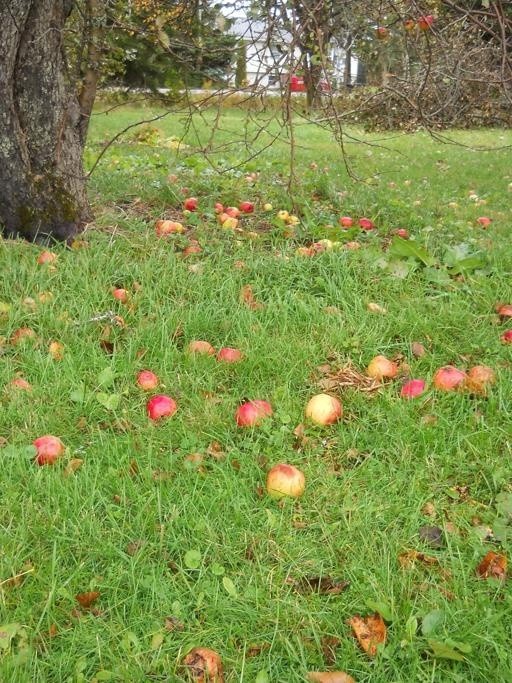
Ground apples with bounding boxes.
[375,26,387,39]
[417,15,433,30]
[403,20,416,31]
[0,160,512,501]
